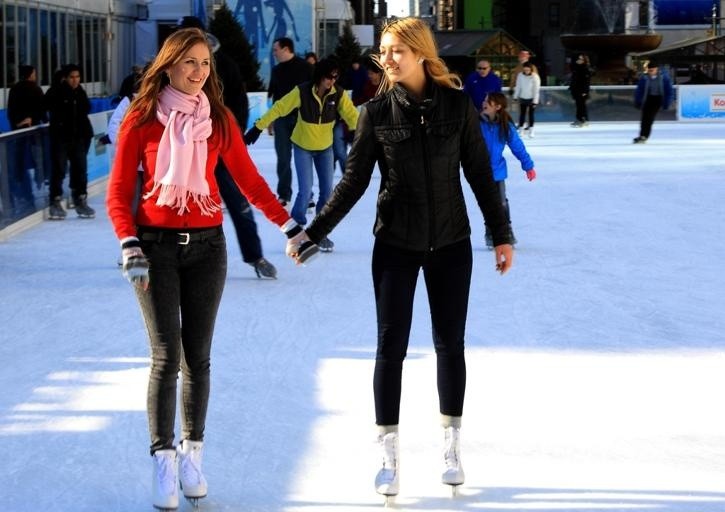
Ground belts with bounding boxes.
[139,227,224,245]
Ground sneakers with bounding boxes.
[633,135,646,142]
[176,439,209,499]
[374,431,401,496]
[571,120,588,126]
[316,232,334,248]
[253,257,276,277]
[516,124,535,132]
[275,198,315,209]
[440,427,467,486]
[48,200,67,216]
[150,449,180,510]
[484,232,518,247]
[73,198,95,215]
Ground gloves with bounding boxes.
[244,126,262,145]
[286,228,319,266]
[527,168,536,182]
[122,247,149,282]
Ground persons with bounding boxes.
[476,91,538,245]
[290,13,518,497]
[174,14,279,279]
[305,52,382,103]
[16,61,99,218]
[568,54,589,128]
[267,35,315,208]
[107,86,147,267]
[108,23,311,510]
[633,61,673,143]
[117,64,144,99]
[509,51,541,131]
[463,60,502,109]
[690,64,708,84]
[7,64,46,190]
[244,59,364,248]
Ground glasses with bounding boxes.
[326,74,338,80]
